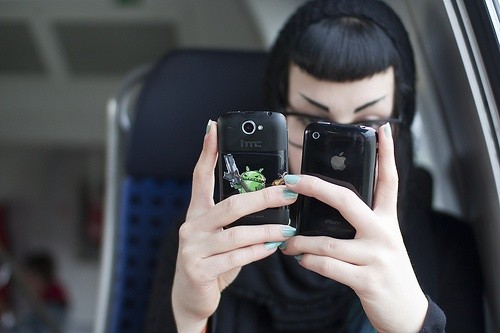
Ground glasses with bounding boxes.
[280,109,403,156]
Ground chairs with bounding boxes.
[87,49,299,333]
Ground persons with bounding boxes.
[147,1,485,333]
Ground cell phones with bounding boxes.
[216,111,292,227]
[297,121,379,239]
